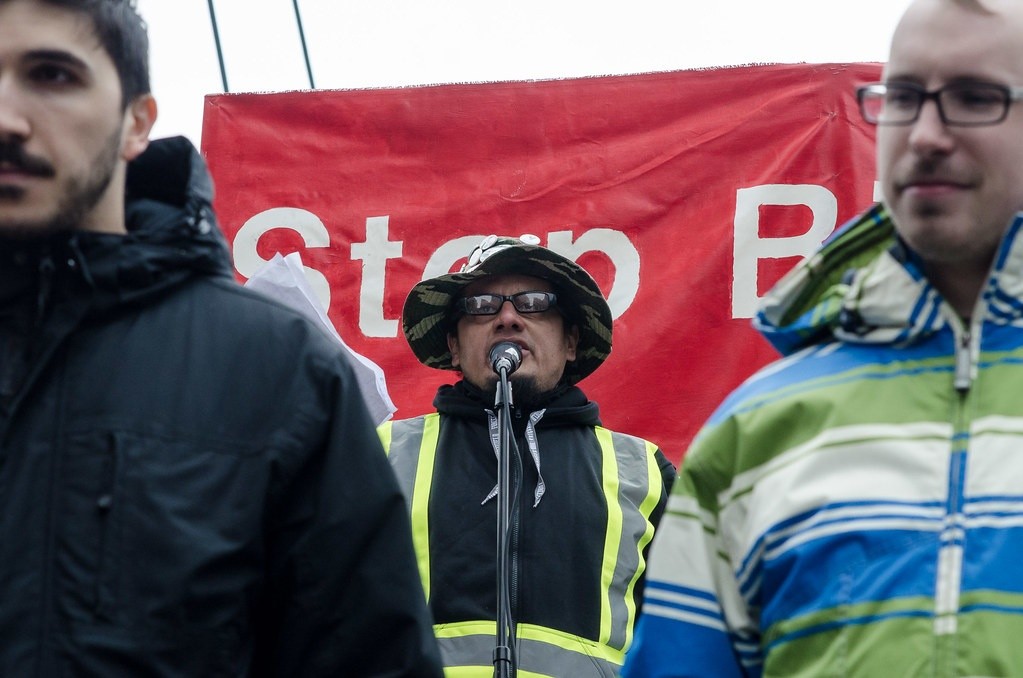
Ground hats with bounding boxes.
[402,240,612,385]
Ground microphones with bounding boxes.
[488,340,525,376]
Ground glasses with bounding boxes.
[855,82,1023,127]
[449,290,570,322]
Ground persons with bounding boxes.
[374,235,678,678]
[618,1,1023,678]
[0,0,450,678]
